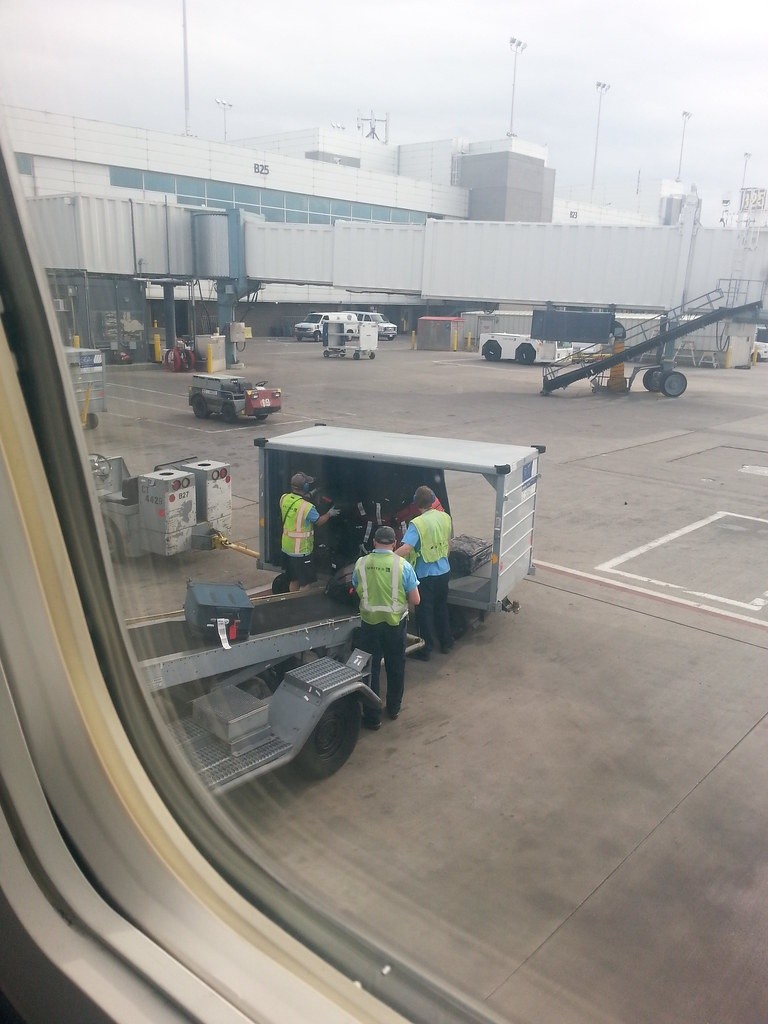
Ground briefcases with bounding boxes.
[450,533,493,573]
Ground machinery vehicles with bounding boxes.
[88,452,233,562]
[189,374,282,423]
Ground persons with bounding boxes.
[395,486,455,660]
[352,526,420,730]
[280,472,340,592]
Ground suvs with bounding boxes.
[295,312,358,342]
[343,311,398,341]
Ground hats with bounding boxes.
[291,472,314,488]
[375,526,396,544]
[412,486,434,508]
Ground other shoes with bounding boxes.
[361,713,381,730]
[442,635,454,655]
[416,649,430,661]
[390,710,400,720]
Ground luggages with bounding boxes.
[186,579,254,641]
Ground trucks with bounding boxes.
[63,346,109,429]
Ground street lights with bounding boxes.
[215,98,232,144]
[330,122,346,165]
[740,152,752,210]
[590,81,611,203]
[678,110,693,177]
[510,37,527,133]
